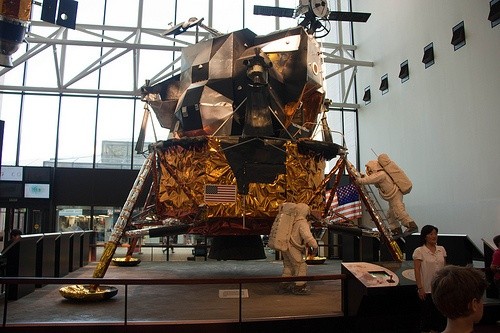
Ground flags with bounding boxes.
[204,184,236,202]
[325,183,363,225]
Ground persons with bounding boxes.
[412,225,448,333]
[355,159,418,240]
[279,203,318,296]
[162,235,176,254]
[1,229,22,254]
[431,265,488,333]
[490,235,500,300]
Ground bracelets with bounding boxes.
[417,287,424,291]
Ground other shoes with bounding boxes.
[404,221,418,237]
[391,227,404,240]
[295,285,312,295]
[280,282,293,293]
